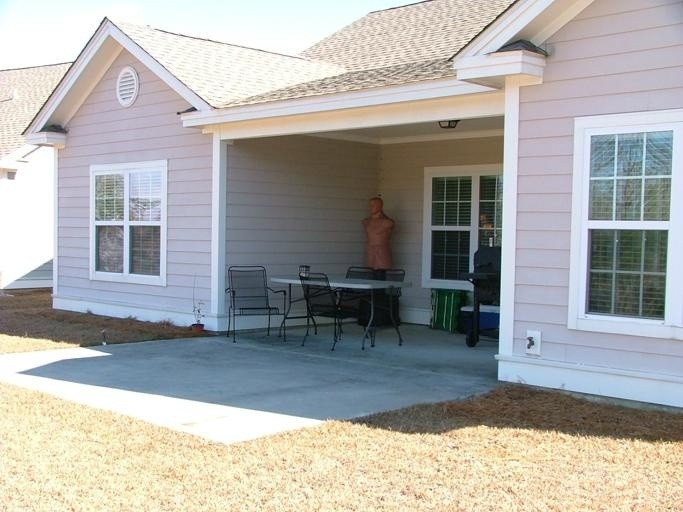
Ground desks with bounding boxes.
[269,274,415,353]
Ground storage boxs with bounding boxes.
[459,304,500,334]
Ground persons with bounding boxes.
[360,197,394,269]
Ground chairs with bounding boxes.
[224,263,407,351]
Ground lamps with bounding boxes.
[438,120,460,128]
